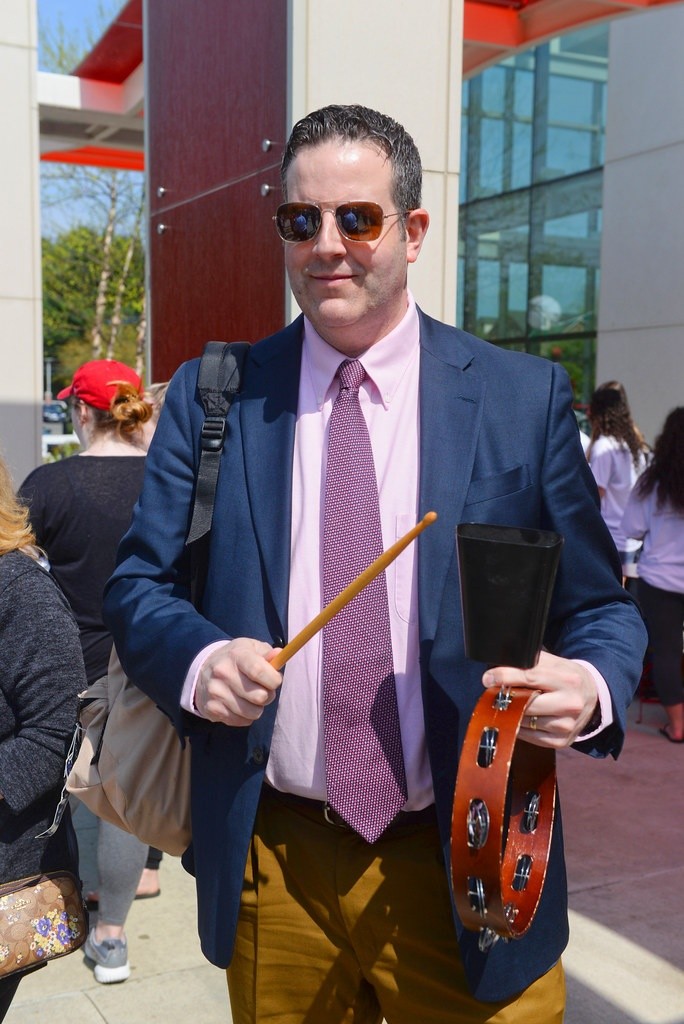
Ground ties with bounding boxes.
[322,360,409,844]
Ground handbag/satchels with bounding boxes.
[0,870,89,981]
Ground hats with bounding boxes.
[597,380,626,407]
[57,359,145,412]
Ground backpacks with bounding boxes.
[65,340,252,856]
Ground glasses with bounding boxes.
[271,201,415,244]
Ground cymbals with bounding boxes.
[446,681,558,953]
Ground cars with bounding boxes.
[44,404,68,421]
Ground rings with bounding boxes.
[530,716,538,730]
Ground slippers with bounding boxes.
[84,884,161,912]
[659,723,684,743]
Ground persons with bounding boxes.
[288,208,317,240]
[339,207,368,234]
[585,381,654,590]
[0,456,86,1024]
[85,381,170,908]
[15,359,152,983]
[99,104,648,1024]
[620,407,684,745]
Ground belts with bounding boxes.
[262,780,351,830]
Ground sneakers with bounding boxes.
[84,927,131,983]
